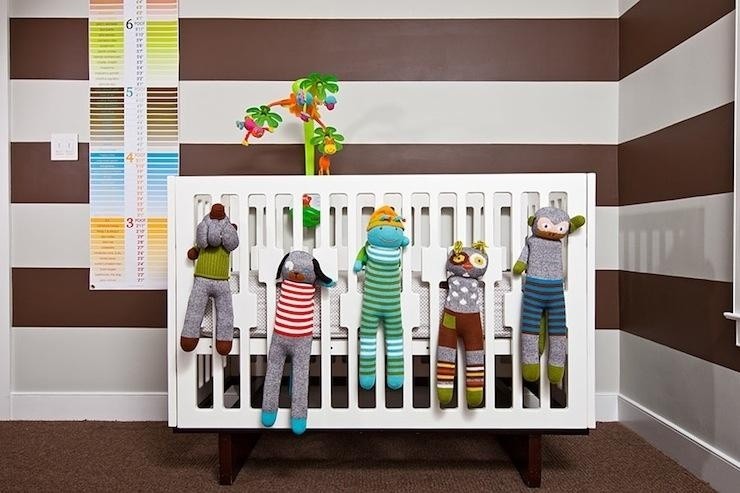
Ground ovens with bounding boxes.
[166,172,594,486]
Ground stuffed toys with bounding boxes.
[354,206,409,389]
[179,203,239,355]
[436,239,489,407]
[262,250,336,433]
[513,207,584,384]
